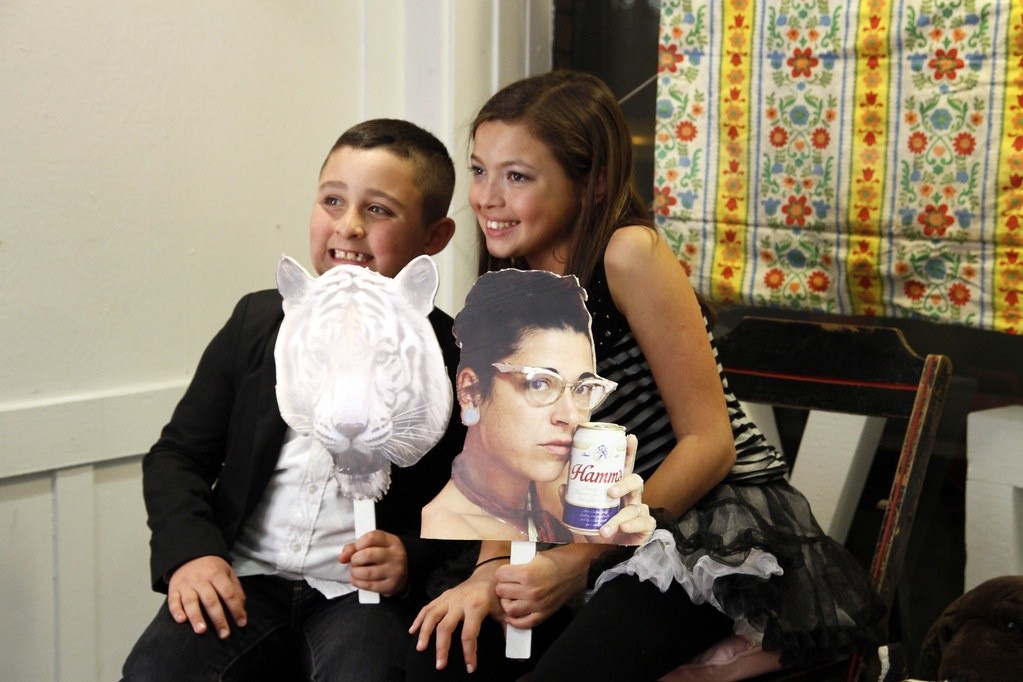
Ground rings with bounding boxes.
[628,504,640,517]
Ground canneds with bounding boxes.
[560,422,627,536]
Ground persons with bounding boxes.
[420,267,656,545]
[405,71,881,682]
[122,119,468,682]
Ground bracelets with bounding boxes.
[474,555,510,570]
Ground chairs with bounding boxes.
[707,313,955,682]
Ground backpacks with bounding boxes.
[902,576,1023,682]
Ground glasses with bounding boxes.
[493,362,618,415]
